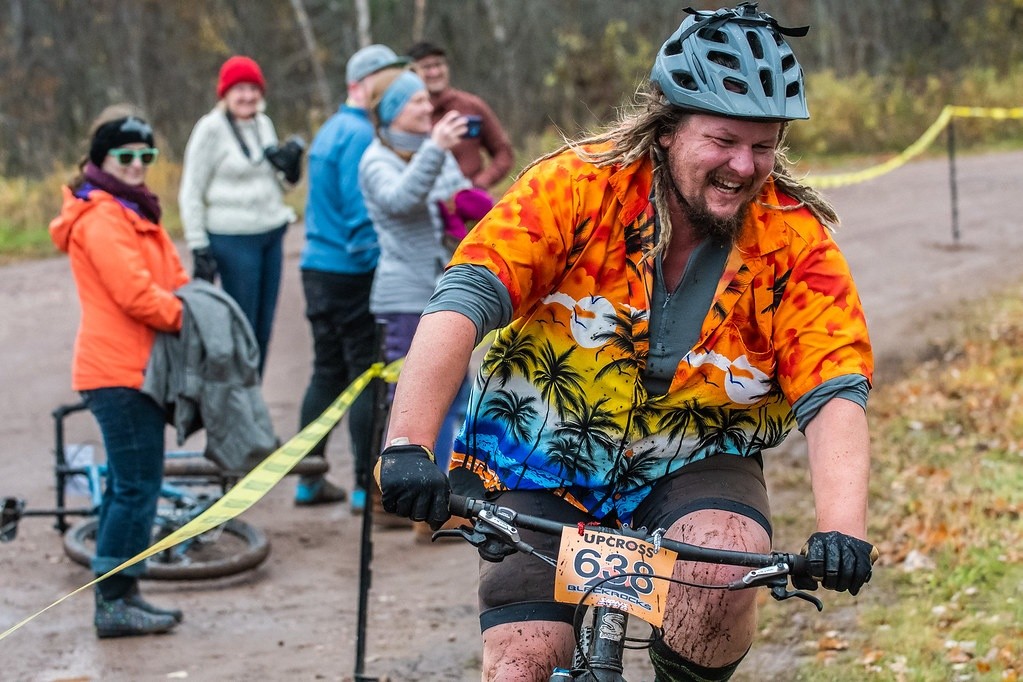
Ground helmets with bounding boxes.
[648,2,812,122]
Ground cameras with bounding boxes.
[453,114,481,138]
[265,140,303,185]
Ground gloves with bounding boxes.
[194,246,218,283]
[374,444,451,531]
[790,531,874,596]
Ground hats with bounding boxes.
[406,41,446,61]
[90,105,155,165]
[217,57,265,95]
[345,44,410,89]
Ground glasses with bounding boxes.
[108,148,159,165]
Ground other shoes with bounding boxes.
[295,478,345,505]
[351,485,367,513]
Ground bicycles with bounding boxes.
[427,492,829,682]
[0,400,331,582]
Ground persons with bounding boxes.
[70,104,189,636]
[177,57,305,388]
[295,42,518,548]
[371,9,874,681]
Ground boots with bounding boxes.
[124,586,182,622]
[94,583,175,638]
